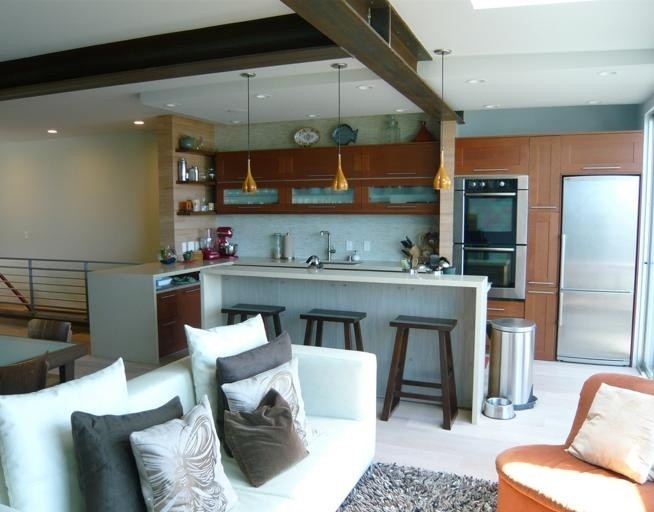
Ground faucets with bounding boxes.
[319,230,336,261]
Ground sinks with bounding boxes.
[302,262,361,265]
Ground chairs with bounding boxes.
[28,318,72,343]
[1,351,48,395]
[495,372,653,512]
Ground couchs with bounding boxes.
[0,342,376,511]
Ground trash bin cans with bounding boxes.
[486,318,538,411]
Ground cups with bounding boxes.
[184,254,191,260]
[410,269,417,278]
[434,271,443,277]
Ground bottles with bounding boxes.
[283,232,294,258]
[272,232,283,259]
[179,198,214,212]
[384,119,400,144]
[179,157,215,182]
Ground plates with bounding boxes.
[294,127,320,147]
[330,124,359,145]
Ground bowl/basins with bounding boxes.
[180,138,194,149]
[483,396,515,420]
[443,267,456,274]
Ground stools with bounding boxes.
[380,316,458,430]
[300,309,367,351]
[221,303,286,337]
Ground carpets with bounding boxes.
[336,463,498,511]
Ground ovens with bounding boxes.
[452,174,528,300]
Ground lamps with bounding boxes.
[433,48,452,190]
[330,62,349,192]
[240,72,257,193]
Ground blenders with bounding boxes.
[217,226,239,259]
[199,225,221,259]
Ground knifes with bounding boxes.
[401,236,412,249]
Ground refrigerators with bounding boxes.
[556,175,640,366]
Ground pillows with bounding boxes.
[563,382,654,486]
[0,357,240,512]
[184,314,312,488]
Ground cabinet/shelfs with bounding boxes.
[157,283,200,359]
[215,141,440,214]
[171,147,215,216]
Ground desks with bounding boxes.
[0,334,88,383]
